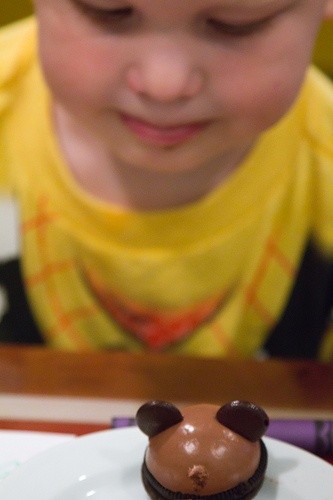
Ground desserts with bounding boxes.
[136,397,270,500]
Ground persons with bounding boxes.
[0,0,333,361]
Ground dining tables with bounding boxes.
[0,341,333,500]
